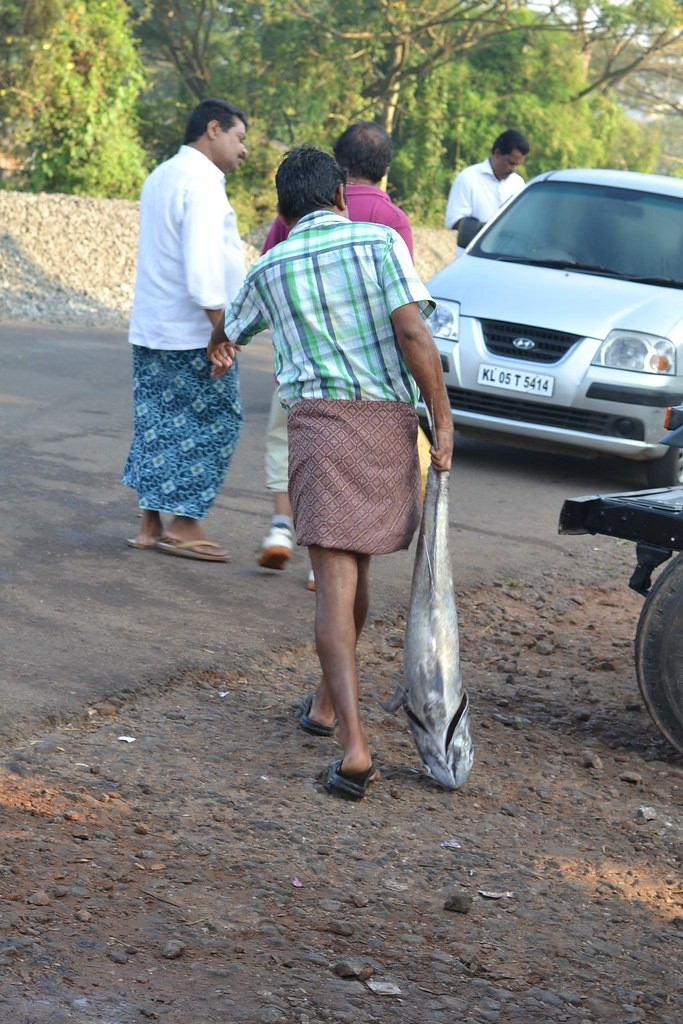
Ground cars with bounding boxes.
[411,167,683,491]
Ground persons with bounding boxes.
[119,97,248,561]
[257,122,413,589]
[207,144,453,800]
[446,129,530,260]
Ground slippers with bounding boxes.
[327,758,377,797]
[299,691,338,738]
[156,540,230,560]
[127,534,168,549]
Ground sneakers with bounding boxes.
[307,569,316,591]
[256,527,292,570]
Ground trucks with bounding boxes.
[556,404,683,757]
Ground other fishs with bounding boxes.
[378,395,475,789]
